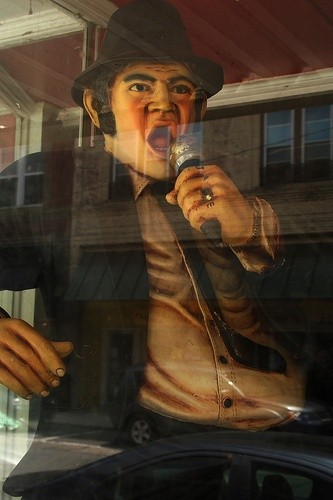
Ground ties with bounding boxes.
[148,180,288,375]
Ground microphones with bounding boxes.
[166,135,224,247]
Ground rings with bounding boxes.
[200,187,216,204]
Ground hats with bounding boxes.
[71,0,224,110]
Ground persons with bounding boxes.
[1,2,332,500]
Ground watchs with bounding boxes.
[232,197,262,247]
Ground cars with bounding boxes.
[19,431,333,500]
[112,365,333,447]
